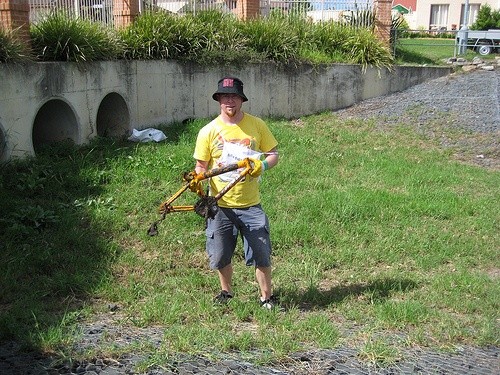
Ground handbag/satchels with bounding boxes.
[217,141,263,183]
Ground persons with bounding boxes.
[192,76,288,312]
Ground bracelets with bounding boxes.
[262,160,268,171]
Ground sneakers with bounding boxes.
[214,289,235,307]
[259,293,286,314]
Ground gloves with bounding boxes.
[248,157,268,178]
[183,178,204,195]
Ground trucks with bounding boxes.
[456,29,500,56]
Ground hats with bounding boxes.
[212,76,248,102]
